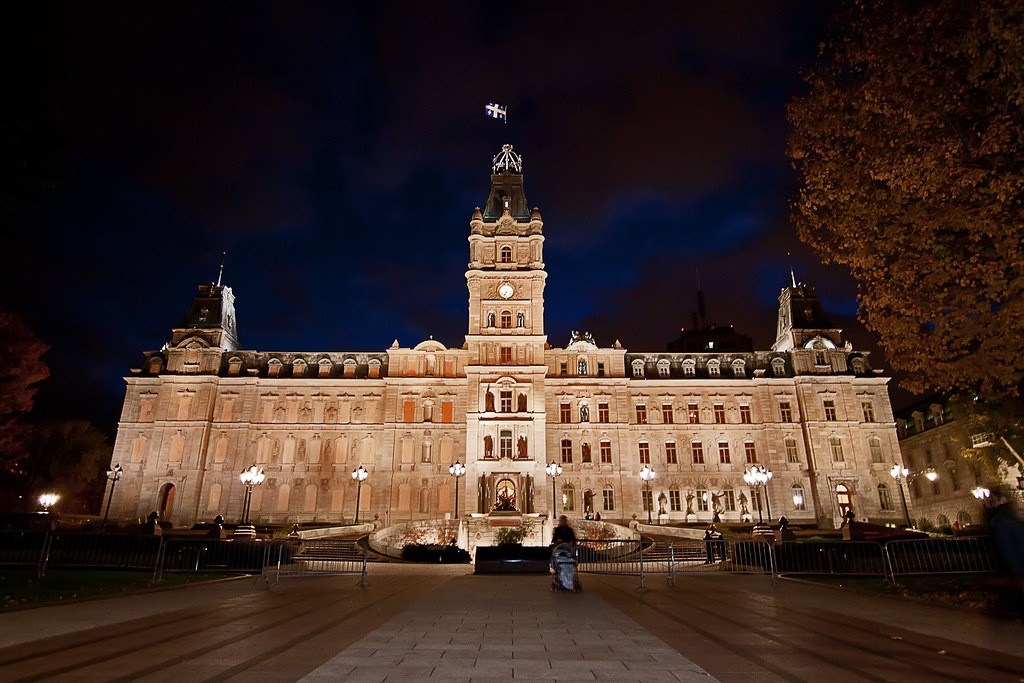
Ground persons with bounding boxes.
[658,492,668,514]
[686,491,695,513]
[551,515,576,546]
[594,512,600,521]
[703,530,715,564]
[712,493,726,512]
[739,493,749,514]
[951,519,959,529]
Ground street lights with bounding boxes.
[545,460,562,520]
[744,464,774,524]
[448,458,467,520]
[890,462,913,530]
[351,465,370,524]
[240,465,266,525]
[102,463,124,529]
[640,465,657,524]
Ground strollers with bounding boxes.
[550,542,583,593]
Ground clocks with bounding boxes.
[499,285,514,299]
